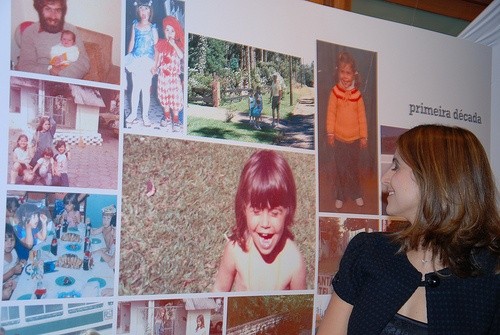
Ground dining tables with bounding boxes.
[10,225,114,300]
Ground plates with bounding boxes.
[55,275,76,286]
[17,293,33,300]
[41,244,52,251]
[47,230,54,236]
[69,227,79,232]
[87,278,106,288]
[92,238,101,244]
[64,244,81,252]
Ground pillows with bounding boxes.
[82,39,105,82]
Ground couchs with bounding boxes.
[18,24,121,85]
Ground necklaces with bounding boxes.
[414,242,440,266]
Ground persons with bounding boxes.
[10,115,71,187]
[249,85,263,131]
[212,150,308,292]
[313,123,495,335]
[18,0,90,82]
[84,205,116,298]
[59,193,82,228]
[123,0,185,132]
[327,53,369,208]
[267,74,282,127]
[212,78,221,107]
[1,195,48,302]
[192,313,207,335]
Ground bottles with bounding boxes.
[36,249,43,279]
[85,222,91,236]
[56,225,60,239]
[62,219,67,232]
[51,239,57,256]
[83,252,89,271]
[84,237,90,252]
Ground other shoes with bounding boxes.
[173,123,180,132]
[143,118,151,126]
[336,200,343,208]
[126,114,138,122]
[355,197,364,206]
[161,118,171,127]
[250,119,279,130]
